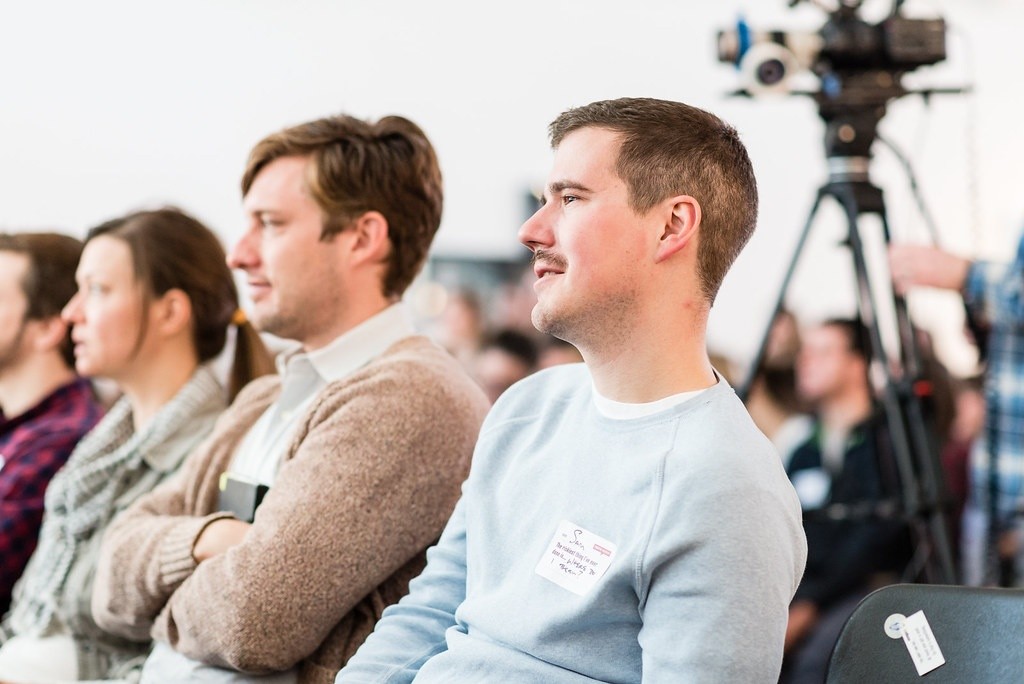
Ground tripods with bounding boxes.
[735,106,956,590]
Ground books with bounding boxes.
[219,475,270,523]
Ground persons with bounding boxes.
[0,209,279,684]
[892,224,1023,582]
[746,292,985,577]
[336,99,806,684]
[90,116,490,683]
[0,232,106,613]
[435,301,583,401]
[785,321,903,656]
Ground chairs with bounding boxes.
[826,583,1024,684]
